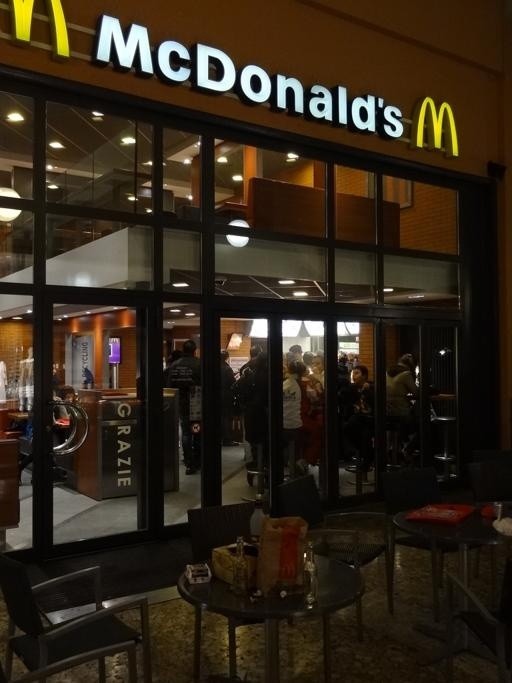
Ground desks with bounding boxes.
[176,550,364,681]
[392,503,511,665]
[5,166,151,258]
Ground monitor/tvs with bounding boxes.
[108,335,122,364]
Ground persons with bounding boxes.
[18,370,80,488]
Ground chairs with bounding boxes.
[396,447,511,621]
[272,472,396,642]
[0,552,154,683]
[446,556,511,682]
[187,502,295,680]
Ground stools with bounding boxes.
[382,421,407,471]
[238,429,312,504]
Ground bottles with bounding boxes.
[250,493,266,544]
[233,536,248,596]
[304,550,320,604]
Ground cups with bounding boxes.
[481,501,496,528]
